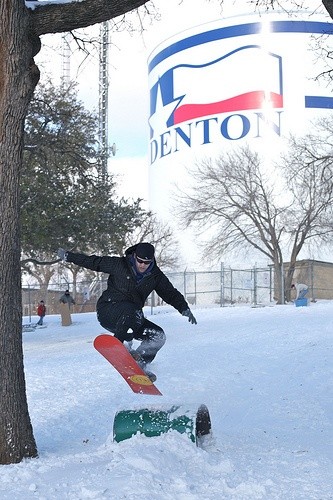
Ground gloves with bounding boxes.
[57,247,68,261]
[182,309,198,325]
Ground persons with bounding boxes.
[36,300,46,325]
[291,283,309,300]
[58,242,197,383]
[59,290,76,310]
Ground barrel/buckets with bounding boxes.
[113,404,211,447]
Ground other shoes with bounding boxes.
[136,357,157,383]
[123,340,141,360]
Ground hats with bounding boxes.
[134,244,154,260]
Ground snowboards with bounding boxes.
[93,335,164,397]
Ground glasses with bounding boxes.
[135,256,152,265]
